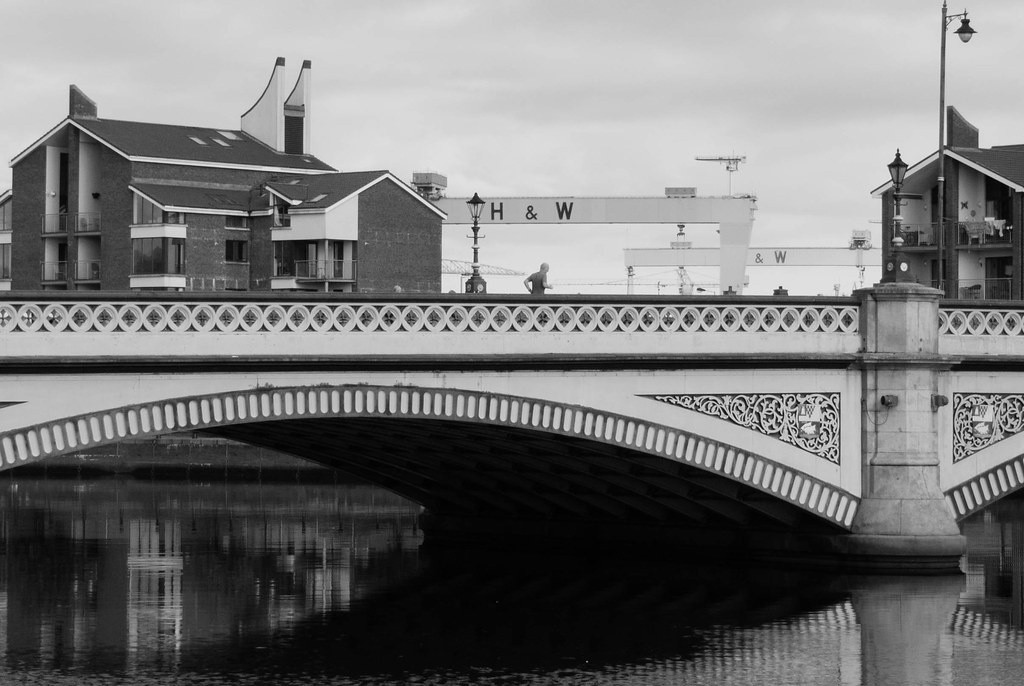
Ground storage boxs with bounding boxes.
[665,187,697,196]
[413,173,447,187]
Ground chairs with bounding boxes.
[962,284,982,299]
[963,222,987,245]
[91,263,98,279]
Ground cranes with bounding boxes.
[694,151,746,198]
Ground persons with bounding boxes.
[524,262,554,295]
[393,285,402,294]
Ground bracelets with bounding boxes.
[529,289,532,291]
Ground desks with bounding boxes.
[987,219,1007,243]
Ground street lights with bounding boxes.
[462,192,487,293]
[886,147,917,282]
[936,1,979,295]
